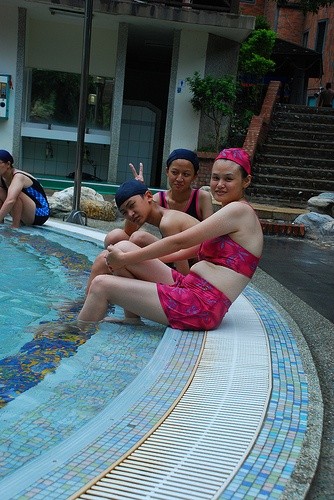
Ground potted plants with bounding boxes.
[185,69,240,189]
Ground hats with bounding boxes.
[215,148,251,175]
[0,150,14,164]
[114,179,148,208]
[166,149,199,175]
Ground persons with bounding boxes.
[0,149,51,228]
[85,179,202,318]
[318,82,334,107]
[104,149,214,249]
[77,148,264,330]
